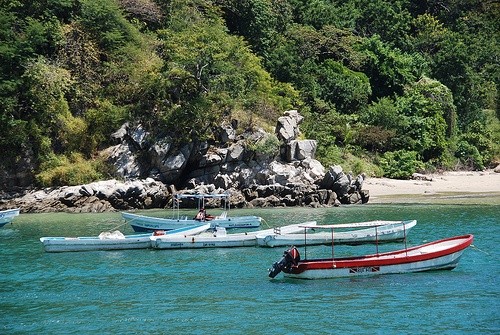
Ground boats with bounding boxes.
[40,222,212,256]
[267,231,475,281]
[254,216,419,248]
[122,193,265,239]
[0,206,23,227]
[148,221,317,251]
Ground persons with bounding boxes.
[196,207,206,221]
